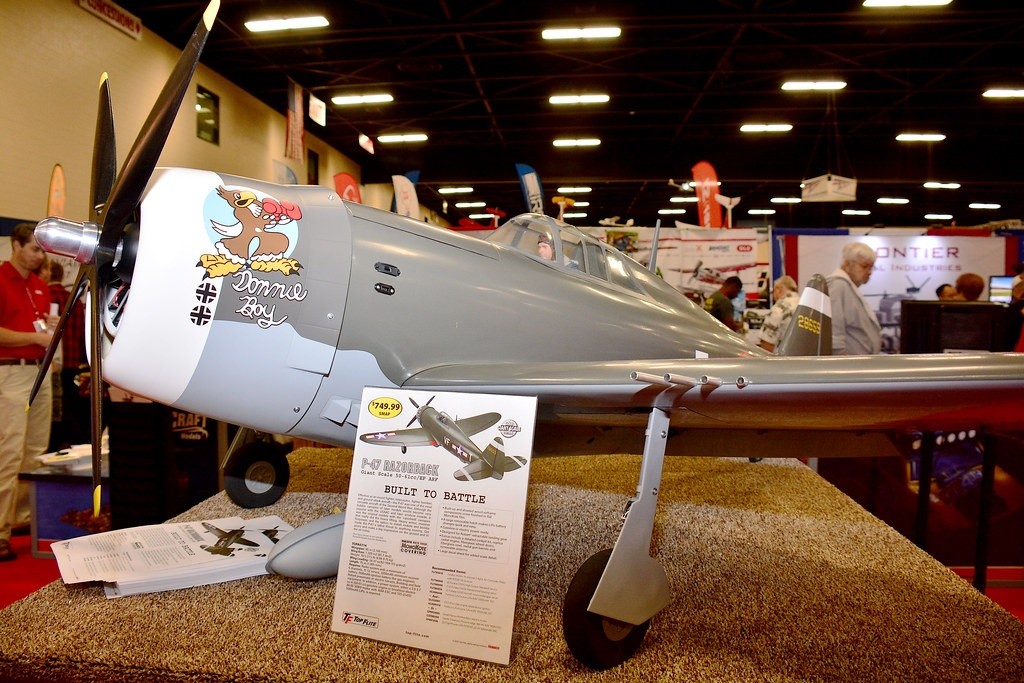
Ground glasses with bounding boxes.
[852,260,876,270]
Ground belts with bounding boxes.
[0,359,45,366]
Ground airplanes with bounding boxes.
[0,0,1024,670]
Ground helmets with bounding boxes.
[537,231,563,261]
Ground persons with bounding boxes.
[757,275,800,352]
[537,232,578,269]
[936,262,1024,353]
[0,223,109,559]
[824,242,882,354]
[702,276,743,332]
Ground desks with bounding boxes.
[0,447,1024,683]
[17,448,111,558]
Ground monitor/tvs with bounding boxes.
[988,275,1015,303]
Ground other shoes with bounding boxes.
[0,539,15,562]
[10,521,31,536]
